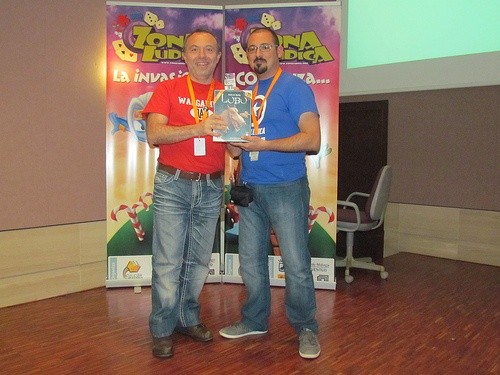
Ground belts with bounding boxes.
[157,163,225,180]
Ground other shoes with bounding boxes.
[177,324,214,341]
[151,336,174,358]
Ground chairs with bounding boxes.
[336,165,393,283]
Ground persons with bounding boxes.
[219,27,321,359]
[141,28,242,358]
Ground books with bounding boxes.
[212,89,252,143]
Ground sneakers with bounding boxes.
[218,321,267,339]
[298,327,321,358]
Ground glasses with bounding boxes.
[246,44,278,54]
